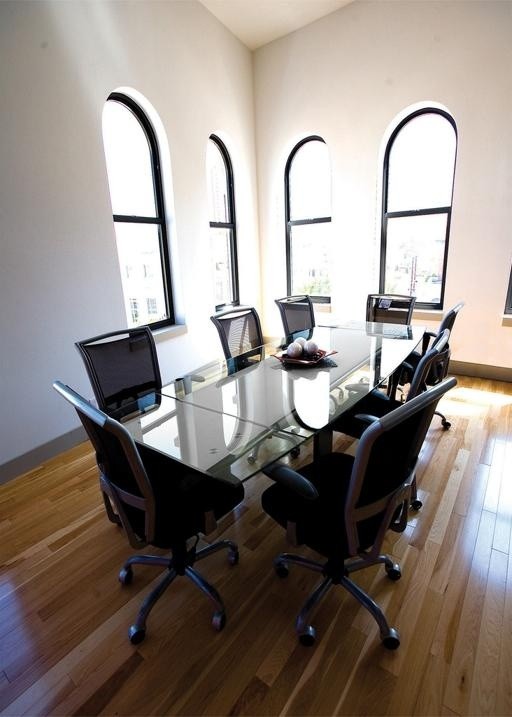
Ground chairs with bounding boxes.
[210,307,264,371]
[73,326,162,412]
[366,294,417,325]
[332,328,450,511]
[53,382,246,644]
[374,303,465,430]
[261,376,455,653]
[274,295,315,335]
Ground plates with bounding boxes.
[269,349,338,364]
